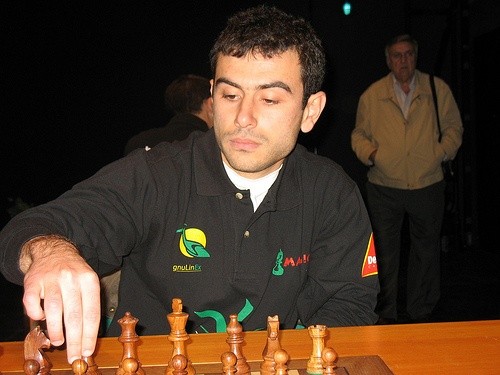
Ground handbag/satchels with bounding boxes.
[441,161,463,216]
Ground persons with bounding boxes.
[125,74,215,155]
[0,7,382,365]
[351,34,465,323]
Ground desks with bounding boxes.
[1,320,500,375]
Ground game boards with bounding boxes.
[2,355,393,375]
